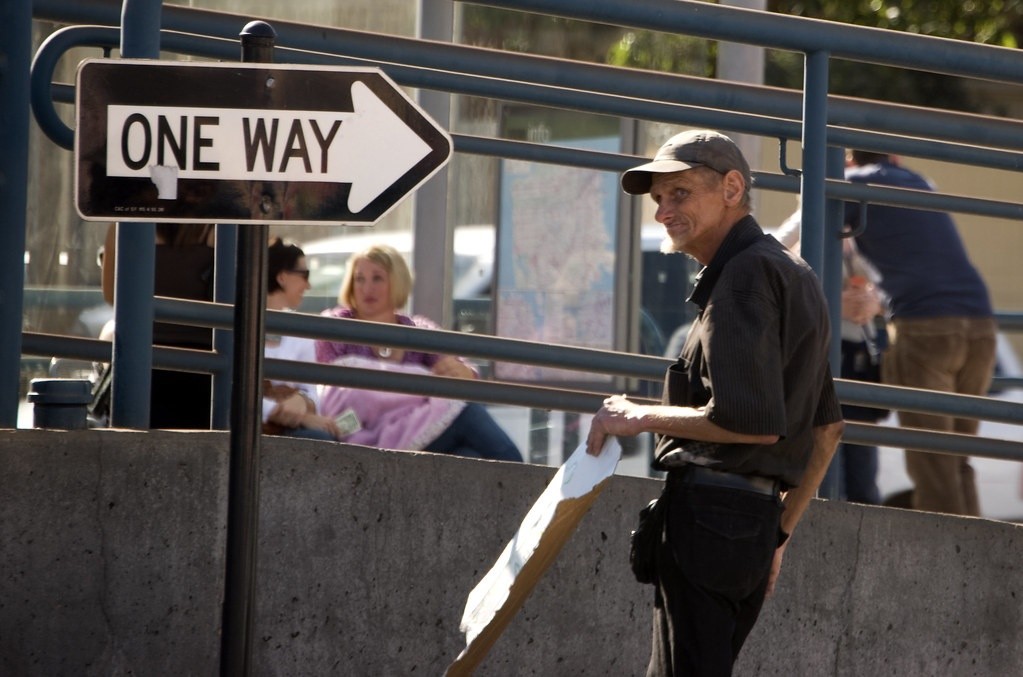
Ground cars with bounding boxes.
[93,218,1023,525]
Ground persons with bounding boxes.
[586,129,849,677]
[93,222,525,461]
[770,148,997,517]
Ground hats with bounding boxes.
[620,130,749,195]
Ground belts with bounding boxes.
[666,468,782,498]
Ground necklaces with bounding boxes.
[378,346,392,358]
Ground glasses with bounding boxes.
[278,267,308,279]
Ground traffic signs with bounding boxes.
[67,56,455,220]
